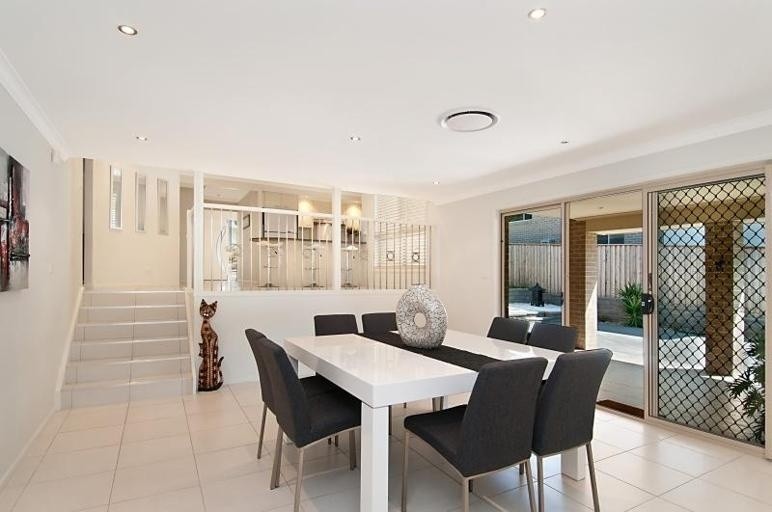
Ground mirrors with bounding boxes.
[110,162,169,239]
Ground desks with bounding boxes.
[281,328,568,511]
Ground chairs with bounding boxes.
[399,355,549,511]
[486,316,531,345]
[361,313,398,332]
[527,321,578,352]
[313,313,359,376]
[519,346,613,512]
[259,336,361,512]
[244,327,334,489]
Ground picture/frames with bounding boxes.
[243,214,250,229]
